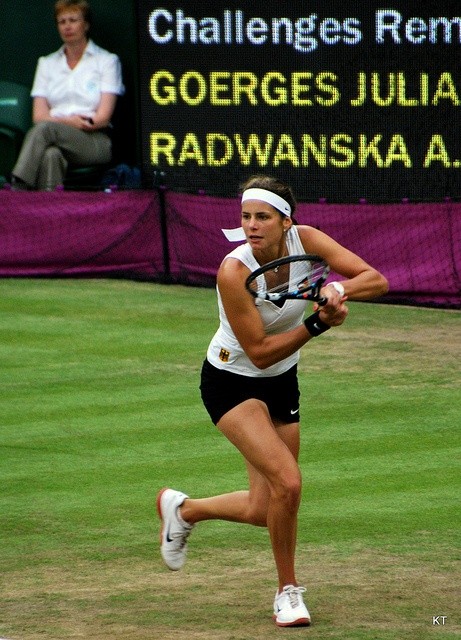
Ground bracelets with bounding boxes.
[328,279,348,298]
[303,309,331,337]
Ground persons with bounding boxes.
[157,173,389,627]
[10,0,126,191]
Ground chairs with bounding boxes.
[1,80,32,190]
[66,121,113,174]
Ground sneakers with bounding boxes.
[273,583,312,628]
[157,487,195,570]
